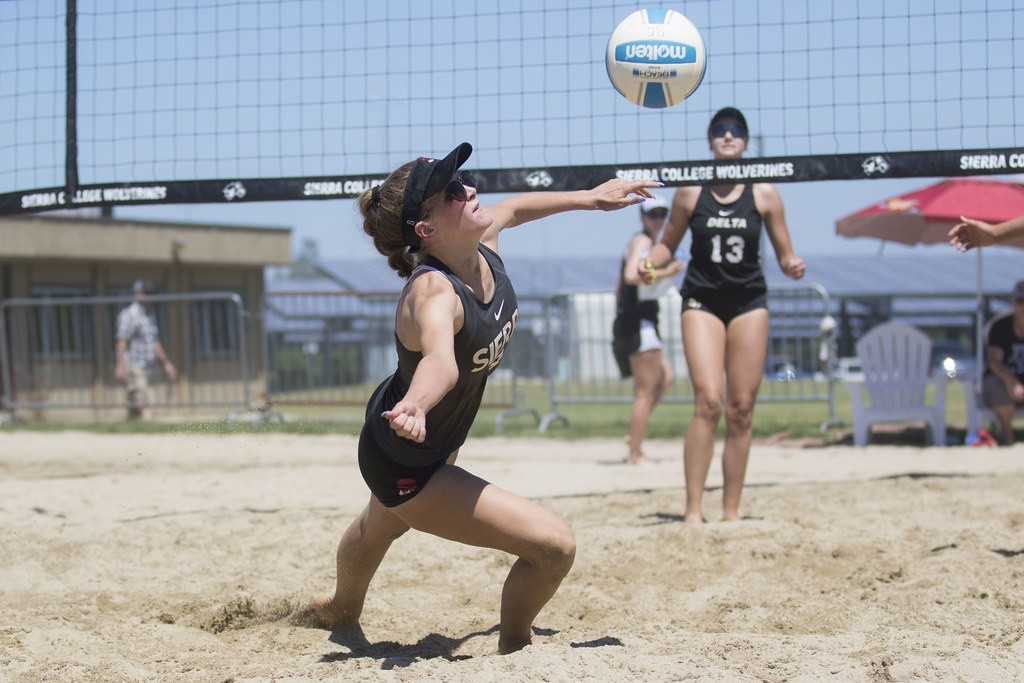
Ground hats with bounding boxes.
[401,142,472,253]
[641,196,669,213]
[708,108,749,135]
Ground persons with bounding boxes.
[983,282,1024,446]
[116,278,178,421]
[313,143,666,656]
[638,107,806,522]
[611,195,688,465]
[948,214,1024,253]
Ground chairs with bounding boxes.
[964,310,1024,444]
[849,322,947,447]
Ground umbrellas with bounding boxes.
[837,181,1024,439]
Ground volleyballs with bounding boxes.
[604,6,709,109]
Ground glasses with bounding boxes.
[412,169,477,241]
[650,210,667,219]
[711,124,745,137]
